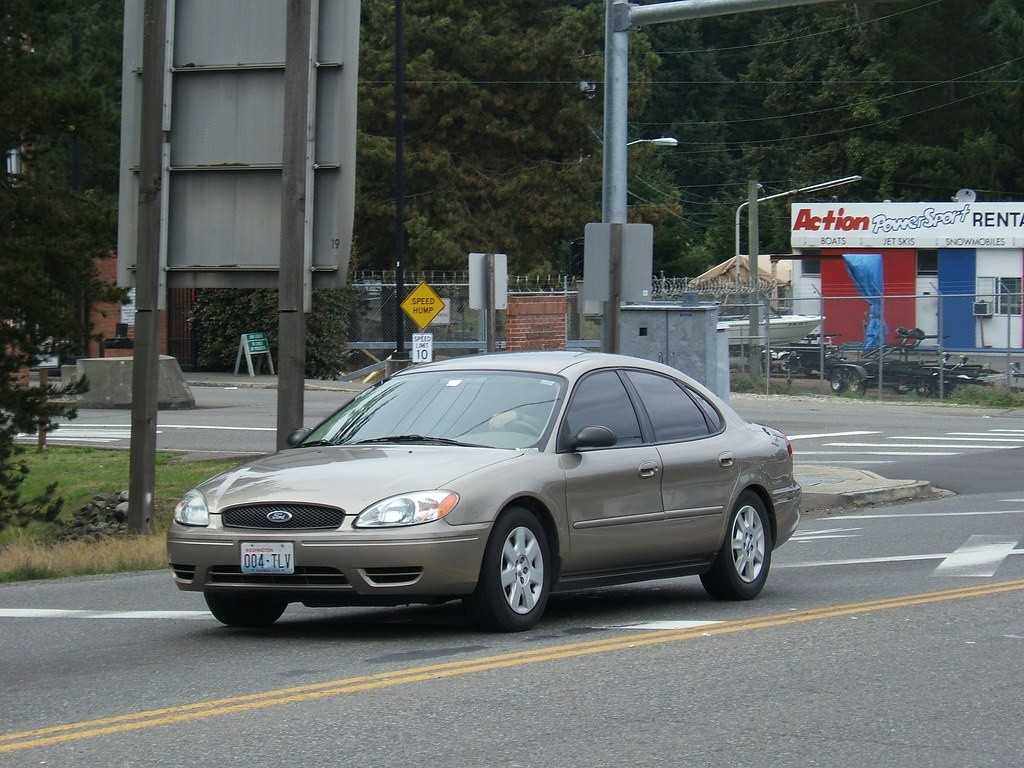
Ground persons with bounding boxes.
[486,379,551,431]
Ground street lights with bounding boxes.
[733,174,863,382]
[623,137,678,151]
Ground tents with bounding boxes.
[685,255,786,299]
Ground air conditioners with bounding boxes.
[973,302,992,316]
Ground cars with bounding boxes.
[32,340,70,369]
[168,352,802,630]
